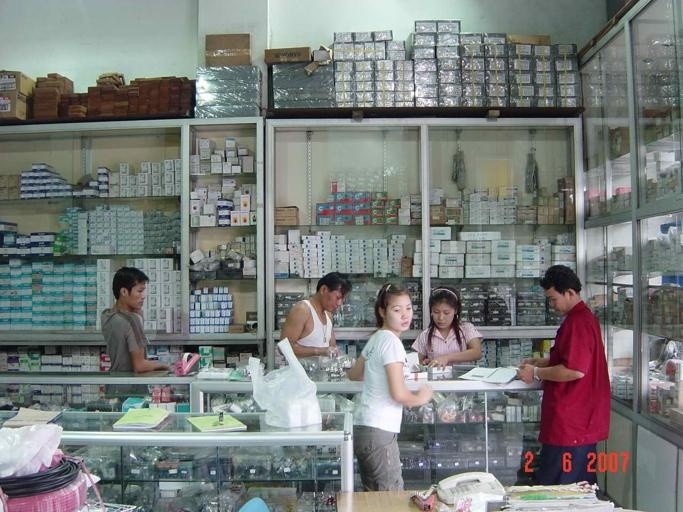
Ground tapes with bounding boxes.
[182,352,193,363]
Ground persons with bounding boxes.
[348,281,434,492]
[278,272,351,360]
[101,267,170,398]
[517,265,611,486]
[411,286,483,371]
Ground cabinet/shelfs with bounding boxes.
[579,0,682,511]
[1,119,265,411]
[1,375,546,512]
[266,115,581,365]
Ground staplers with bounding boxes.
[410,494,437,511]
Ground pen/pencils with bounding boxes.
[418,354,427,366]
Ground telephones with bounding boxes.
[436,471,506,505]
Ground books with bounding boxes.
[185,412,249,434]
[458,366,519,385]
[2,406,64,428]
[110,407,172,431]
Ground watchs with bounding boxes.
[533,367,541,383]
